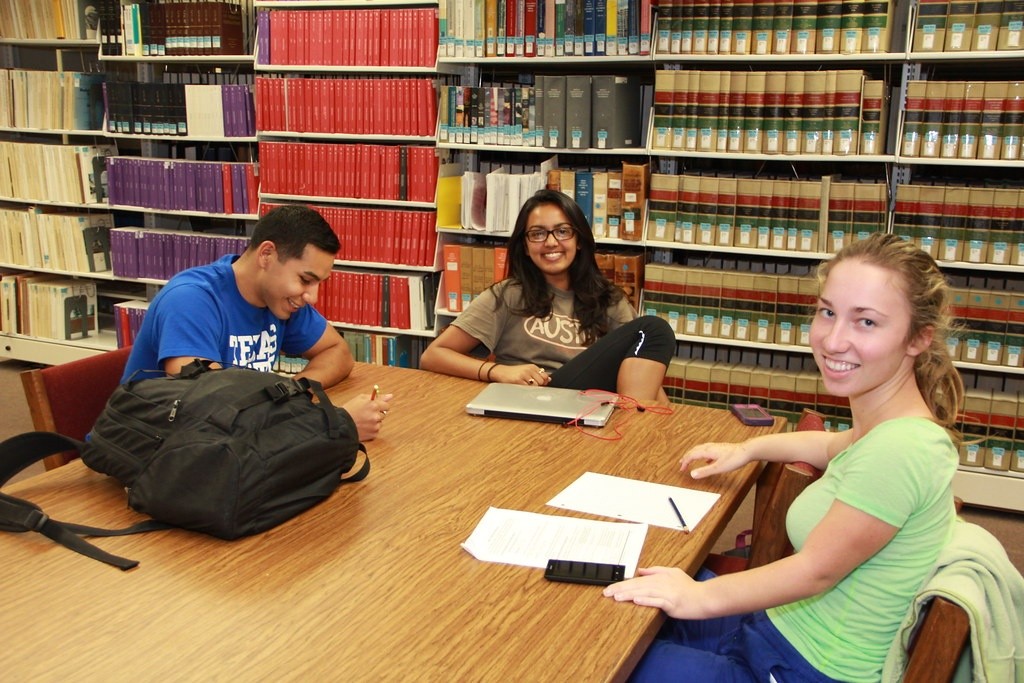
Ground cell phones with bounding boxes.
[731,404,774,426]
[544,560,626,586]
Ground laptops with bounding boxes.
[465,382,620,428]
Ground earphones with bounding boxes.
[637,405,645,412]
[561,422,568,428]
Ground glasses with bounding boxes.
[524,227,575,243]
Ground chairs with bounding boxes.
[881,520,1024,683]
[19,345,133,471]
[704,408,827,576]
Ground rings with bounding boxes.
[539,368,545,375]
[528,379,534,384]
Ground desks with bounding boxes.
[0,362,788,682]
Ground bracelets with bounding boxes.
[478,360,489,381]
[488,363,500,383]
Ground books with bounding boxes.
[2,1,1024,471]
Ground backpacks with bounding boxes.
[0,360,376,570]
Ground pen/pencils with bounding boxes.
[668,497,689,535]
[371,385,379,401]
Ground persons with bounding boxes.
[85,206,392,443]
[419,190,676,402]
[603,233,988,683]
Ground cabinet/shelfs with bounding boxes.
[254,0,441,369]
[437,0,655,324]
[641,0,1023,514]
[0,0,258,366]
